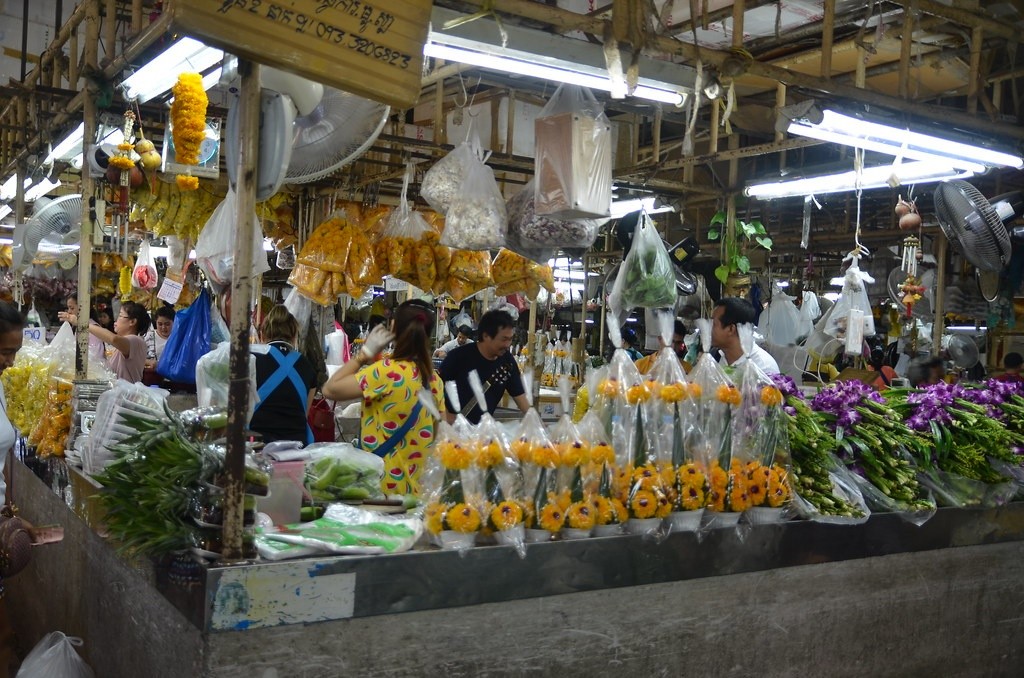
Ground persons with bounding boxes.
[321,298,447,496]
[961,344,985,380]
[996,352,1024,384]
[712,297,780,378]
[868,349,899,390]
[433,324,475,358]
[248,304,318,451]
[634,319,693,376]
[436,308,530,424]
[796,282,834,326]
[0,299,66,678]
[144,299,176,369]
[324,308,350,365]
[35,287,152,383]
[622,329,644,362]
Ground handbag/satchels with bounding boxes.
[0,509,31,577]
[195,179,271,284]
[309,399,335,444]
[131,238,158,289]
[155,288,212,386]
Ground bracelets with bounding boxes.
[354,350,369,367]
[360,344,375,359]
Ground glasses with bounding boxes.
[118,314,132,320]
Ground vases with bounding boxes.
[437,508,784,554]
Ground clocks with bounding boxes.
[162,112,221,178]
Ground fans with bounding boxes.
[58,253,77,270]
[947,333,980,370]
[228,62,392,201]
[662,236,722,302]
[887,264,940,322]
[934,180,1024,273]
[24,192,83,261]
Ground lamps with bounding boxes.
[116,36,224,105]
[742,155,973,201]
[421,32,694,110]
[786,107,1023,177]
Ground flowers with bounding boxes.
[421,372,1024,536]
[91,70,284,308]
[1,354,73,461]
[286,205,556,307]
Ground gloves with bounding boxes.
[361,323,395,358]
